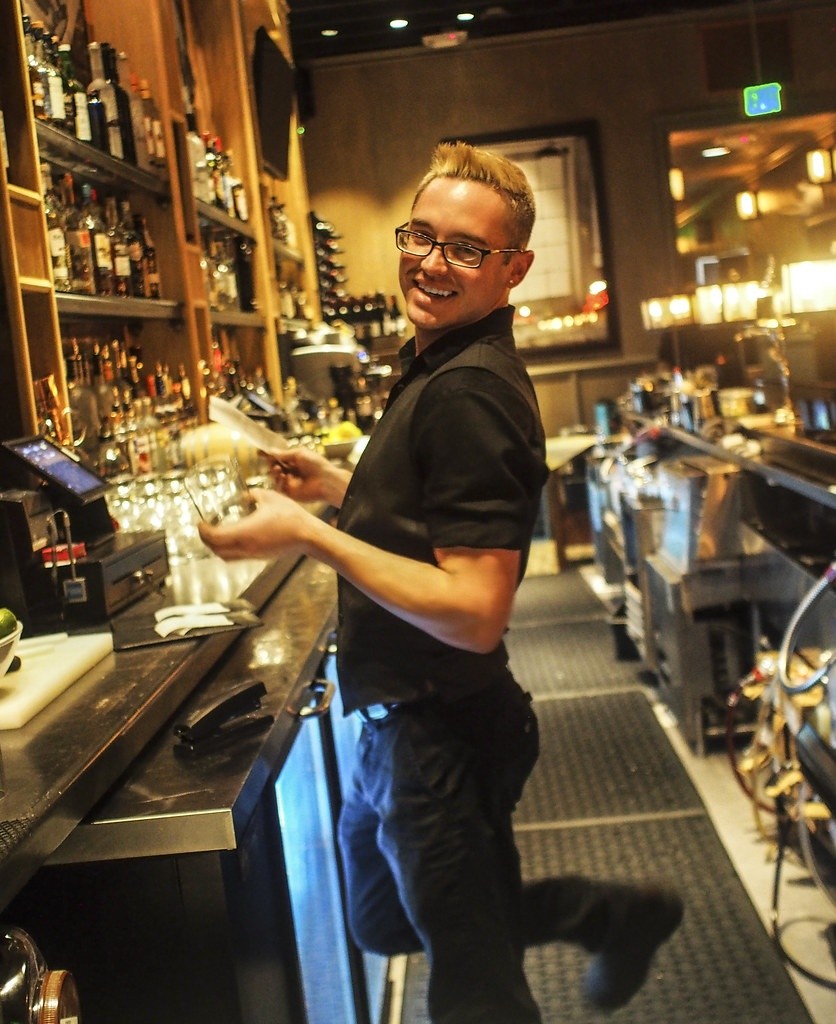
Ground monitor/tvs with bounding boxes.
[1,433,115,506]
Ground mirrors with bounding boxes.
[444,120,624,365]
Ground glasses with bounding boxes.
[394,222,524,269]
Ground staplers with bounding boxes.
[171,674,277,759]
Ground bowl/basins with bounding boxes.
[0,621,24,679]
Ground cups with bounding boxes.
[184,454,257,526]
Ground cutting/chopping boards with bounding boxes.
[0,633,113,729]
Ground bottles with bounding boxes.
[21,11,407,480]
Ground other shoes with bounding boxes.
[584,891,683,1008]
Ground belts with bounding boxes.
[352,702,400,724]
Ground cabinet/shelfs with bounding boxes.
[0,0,321,452]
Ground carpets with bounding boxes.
[399,814,814,1024]
[507,571,605,622]
[513,690,707,824]
[504,619,634,693]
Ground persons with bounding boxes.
[196,138,685,1024]
